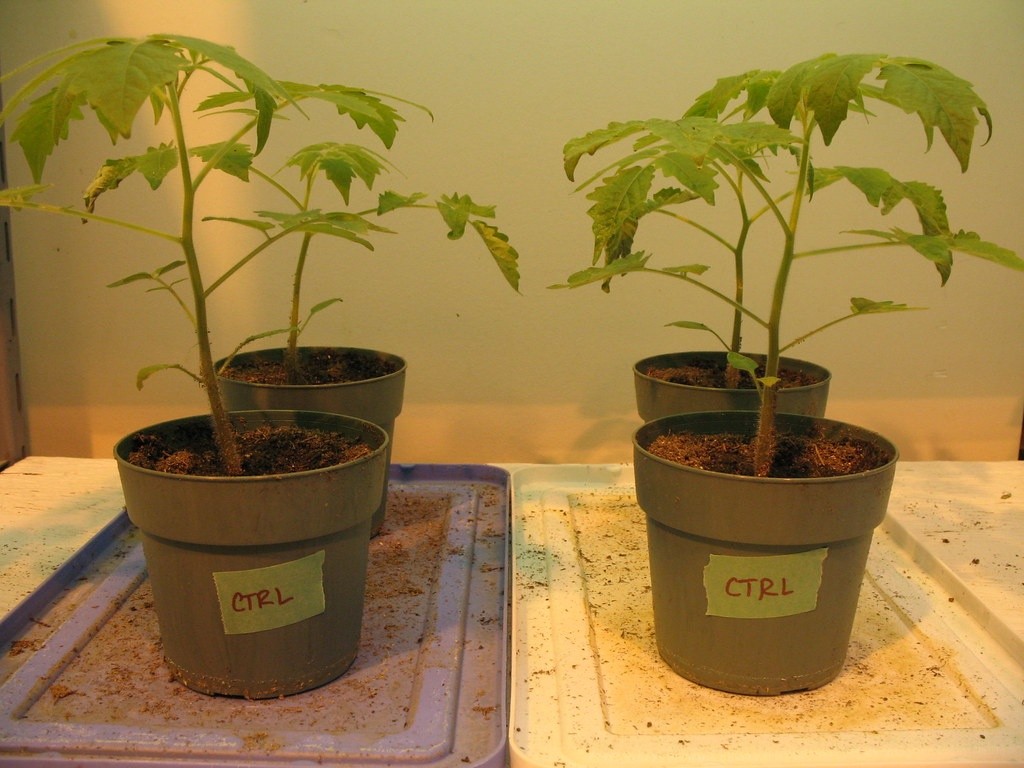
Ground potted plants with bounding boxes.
[0,29,1024,702]
